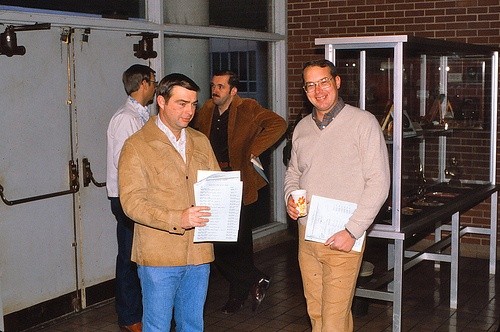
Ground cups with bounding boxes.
[291,189,307,217]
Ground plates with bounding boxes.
[359,260,375,276]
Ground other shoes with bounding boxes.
[359,260,375,276]
[119,320,142,332]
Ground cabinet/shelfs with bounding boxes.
[315,35,500,332]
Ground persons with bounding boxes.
[106,64,158,332]
[189,69,288,313]
[118,73,221,332]
[284,60,390,332]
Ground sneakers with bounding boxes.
[222,286,250,314]
[252,274,272,311]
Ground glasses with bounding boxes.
[302,75,336,94]
[142,78,158,89]
